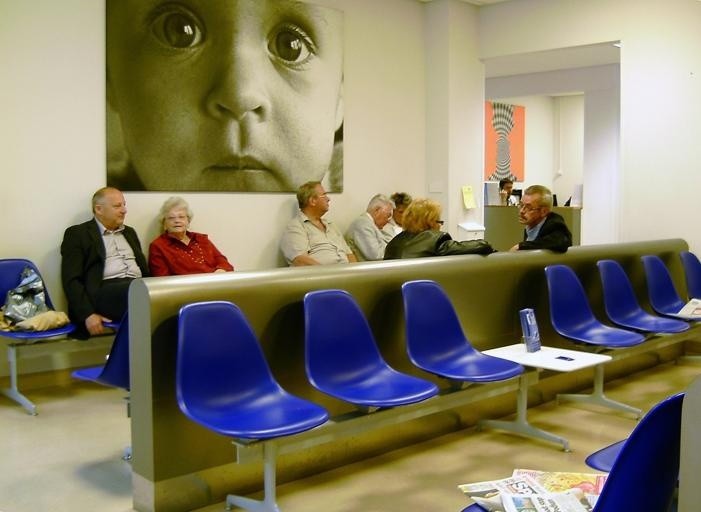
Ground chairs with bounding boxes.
[462,391,685,512]
[595,259,691,334]
[173,299,331,441]
[399,279,522,388]
[73,307,131,470]
[295,287,440,408]
[540,263,646,347]
[639,252,700,322]
[2,257,73,416]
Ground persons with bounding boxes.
[279,178,498,267]
[509,185,573,251]
[498,178,518,207]
[106,0,344,193]
[58,186,150,341]
[147,194,235,277]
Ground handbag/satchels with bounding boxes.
[0,266,71,333]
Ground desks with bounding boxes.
[476,343,612,453]
